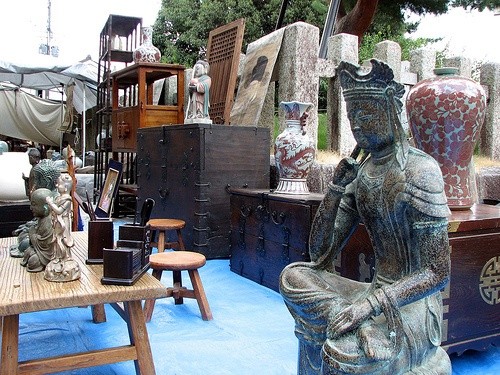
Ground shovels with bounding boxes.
[74,190,96,221]
[140,198,154,226]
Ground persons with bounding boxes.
[278,58,453,375]
[22,148,45,198]
[184,60,213,124]
[20,188,54,273]
[44,173,80,282]
[10,160,61,257]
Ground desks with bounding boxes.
[0,231,168,375]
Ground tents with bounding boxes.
[0,55,120,168]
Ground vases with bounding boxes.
[132,27,161,63]
[272,101,315,194]
[405,67,487,210]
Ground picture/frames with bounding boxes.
[98,167,119,213]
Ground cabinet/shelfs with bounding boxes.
[93,14,143,218]
[110,63,185,219]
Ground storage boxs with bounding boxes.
[229,189,322,294]
[332,204,500,357]
[136,123,271,260]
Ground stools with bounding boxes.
[147,219,185,253]
[143,251,213,323]
